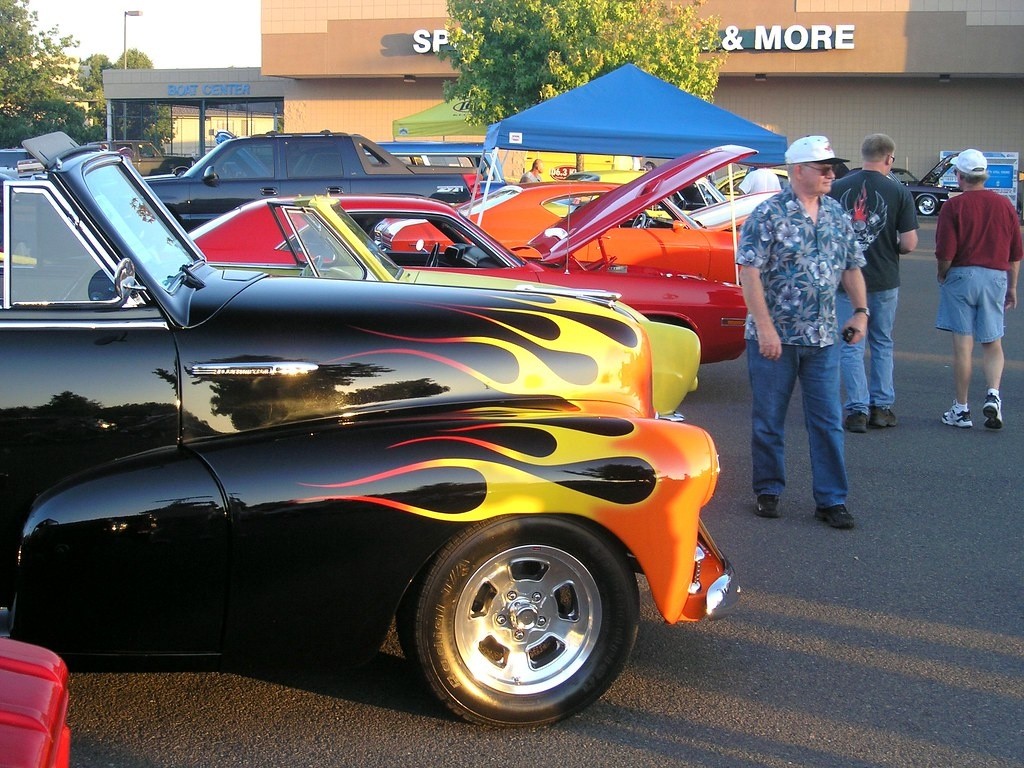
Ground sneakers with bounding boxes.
[869,408,897,427]
[983,393,1003,429]
[753,493,782,518]
[942,407,973,427]
[845,413,869,433]
[814,505,854,528]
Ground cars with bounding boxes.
[1,637,76,768]
[536,169,727,230]
[82,139,195,177]
[0,149,42,182]
[373,173,781,287]
[885,155,959,215]
[702,170,794,201]
[179,144,761,365]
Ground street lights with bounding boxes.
[122,9,144,141]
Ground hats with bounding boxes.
[950,148,987,175]
[784,136,851,164]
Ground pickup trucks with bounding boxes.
[142,128,479,236]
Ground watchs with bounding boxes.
[853,306,870,317]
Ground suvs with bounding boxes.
[0,131,752,727]
[215,130,507,203]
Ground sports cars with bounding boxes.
[206,195,706,428]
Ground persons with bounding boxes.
[738,166,783,195]
[825,132,921,434]
[735,134,872,531]
[934,148,1023,430]
[519,159,546,184]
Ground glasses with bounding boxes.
[802,164,835,176]
[888,155,895,163]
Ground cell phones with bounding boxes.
[843,327,854,344]
